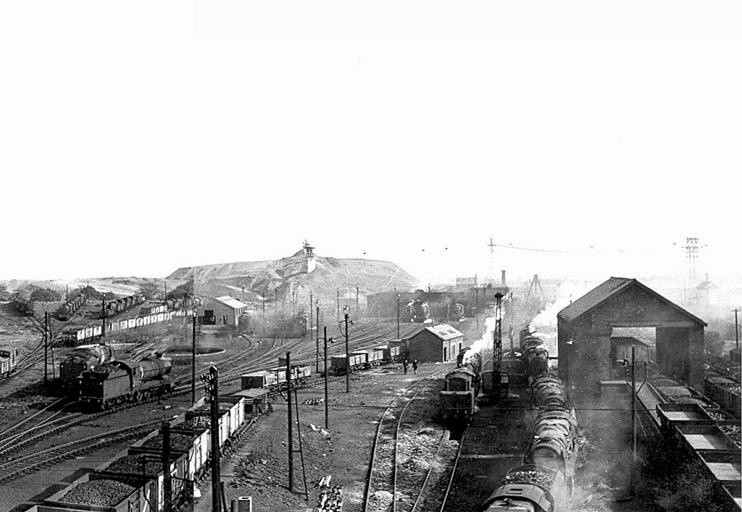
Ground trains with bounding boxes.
[60,344,115,390]
[482,322,578,512]
[0,345,19,376]
[439,350,482,421]
[76,354,171,406]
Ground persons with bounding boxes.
[413,358,418,374]
[403,359,408,374]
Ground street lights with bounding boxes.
[201,366,230,512]
[317,326,336,428]
[277,350,303,492]
[618,345,647,466]
[191,296,204,404]
[262,287,360,335]
[475,287,480,325]
[396,284,436,341]
[338,307,355,395]
